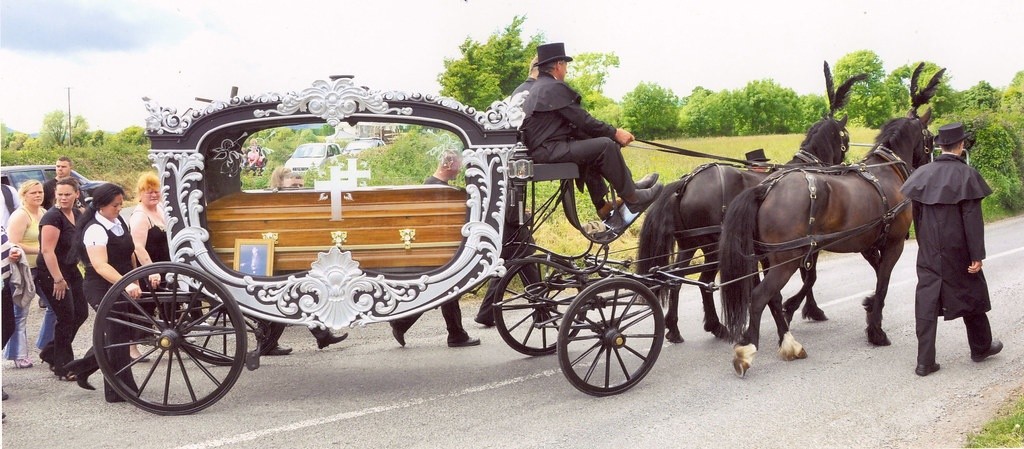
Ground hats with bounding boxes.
[933,123,971,146]
[744,148,770,163]
[533,43,572,66]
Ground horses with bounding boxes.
[636,115,848,346]
[717,106,933,379]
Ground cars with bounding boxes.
[1,164,109,209]
[341,137,386,156]
[284,142,343,174]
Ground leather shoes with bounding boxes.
[972,340,1003,361]
[390,321,406,346]
[447,336,480,347]
[266,347,292,356]
[597,198,624,220]
[317,333,348,349]
[915,363,940,376]
[622,183,664,213]
[634,172,660,188]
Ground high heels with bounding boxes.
[12,356,33,367]
[39,351,78,381]
[64,361,95,391]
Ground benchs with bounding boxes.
[532,162,580,184]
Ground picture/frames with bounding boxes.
[233,238,275,276]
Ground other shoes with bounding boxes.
[473,314,496,327]
[129,354,151,362]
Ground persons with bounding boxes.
[899,123,1003,375]
[388,149,481,347]
[744,149,772,286]
[1,156,348,418]
[509,43,665,220]
[475,181,561,327]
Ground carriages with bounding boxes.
[93,59,947,419]
